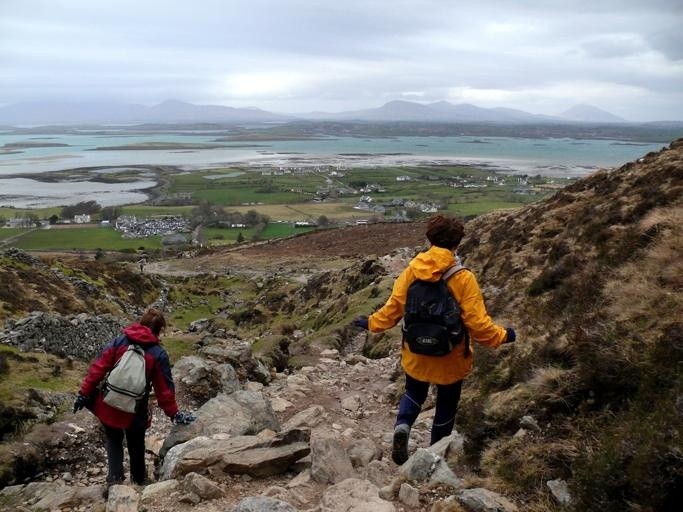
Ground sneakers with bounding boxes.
[391,423,411,465]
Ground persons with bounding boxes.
[353,215,516,467]
[72,309,197,485]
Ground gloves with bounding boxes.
[353,315,368,330]
[506,328,516,342]
[172,409,197,426]
[72,394,85,414]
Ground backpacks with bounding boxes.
[401,265,471,359]
[95,335,158,415]
